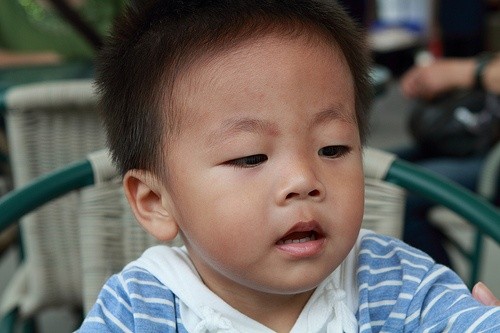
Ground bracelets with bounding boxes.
[469,57,489,88]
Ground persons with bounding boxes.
[74,0,500,333]
[400,1,500,273]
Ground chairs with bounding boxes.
[1,140,500,318]
[0,64,392,333]
